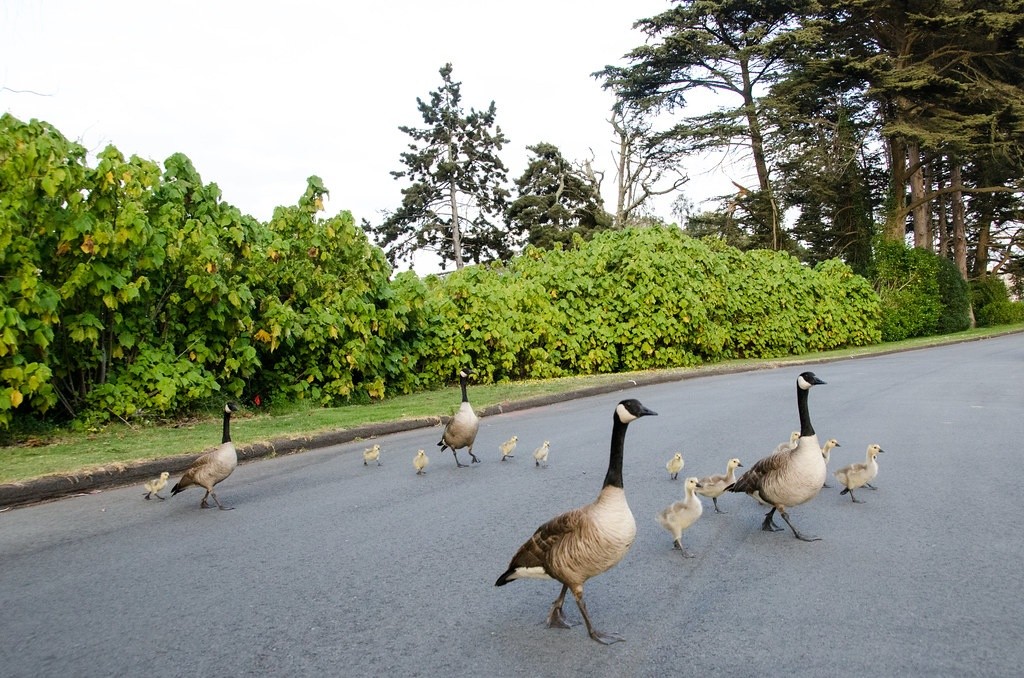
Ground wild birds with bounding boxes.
[143,472,170,500]
[772,431,800,454]
[436,369,481,468]
[820,438,842,489]
[170,402,239,511]
[498,436,519,461]
[362,444,382,466]
[694,458,744,515]
[413,449,429,475]
[494,398,659,645]
[532,440,550,468]
[666,451,685,480]
[722,372,843,543]
[833,444,885,504]
[655,477,703,559]
